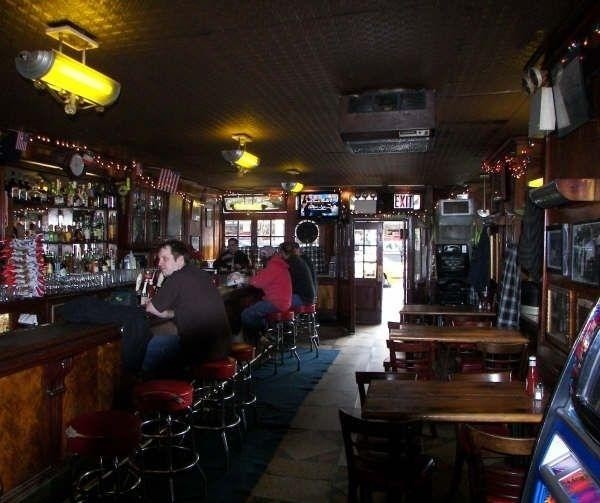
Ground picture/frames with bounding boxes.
[543,217,600,359]
[189,236,200,252]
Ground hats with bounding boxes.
[259,245,275,257]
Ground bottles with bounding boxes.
[525,355,539,395]
[138,270,156,307]
[0,268,144,302]
[534,382,545,401]
[7,171,118,271]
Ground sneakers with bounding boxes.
[257,334,276,352]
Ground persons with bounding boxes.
[130,238,233,374]
[280,239,316,306]
[292,242,317,284]
[212,238,254,270]
[233,245,292,343]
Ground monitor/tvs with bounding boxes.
[299,191,341,219]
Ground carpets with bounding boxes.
[19,344,340,502]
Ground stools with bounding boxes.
[184,354,241,470]
[224,339,260,426]
[288,304,321,358]
[63,411,144,501]
[264,310,301,373]
[127,378,205,501]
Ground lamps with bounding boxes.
[280,168,304,194]
[221,133,261,170]
[16,25,122,115]
[519,68,551,96]
[229,196,269,213]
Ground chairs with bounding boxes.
[338,319,554,503]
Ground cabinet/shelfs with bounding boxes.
[0,158,170,326]
